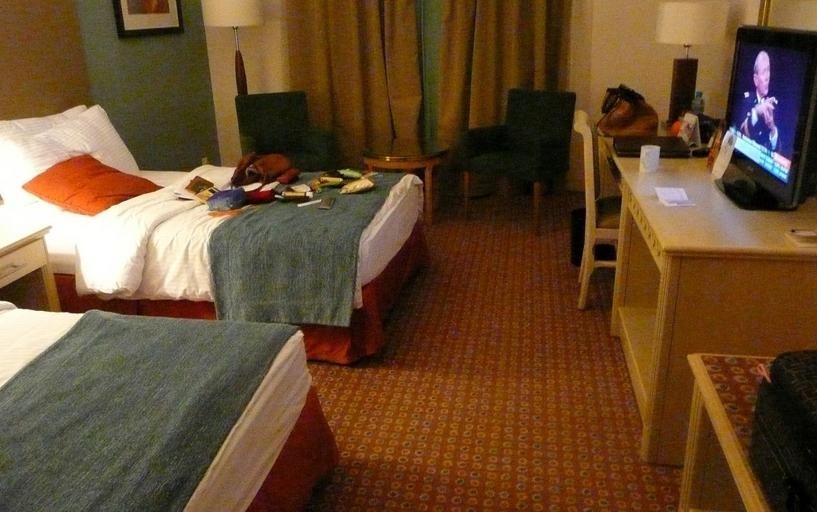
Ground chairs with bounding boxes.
[234,87,351,172]
[456,84,577,214]
[566,105,626,314]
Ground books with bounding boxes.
[784,228,816,248]
[172,173,222,204]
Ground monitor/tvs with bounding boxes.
[713,25,816,212]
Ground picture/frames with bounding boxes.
[112,0,189,38]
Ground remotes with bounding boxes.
[318,197,336,210]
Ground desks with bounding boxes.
[362,138,456,226]
[595,127,817,469]
[670,348,782,512]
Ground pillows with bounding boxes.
[0,105,89,145]
[0,105,139,198]
[25,155,159,218]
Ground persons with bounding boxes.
[731,50,790,153]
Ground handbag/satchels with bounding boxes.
[595,84,658,137]
[749,378,815,512]
[231,152,290,187]
[770,349,816,422]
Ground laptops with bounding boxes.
[612,134,690,158]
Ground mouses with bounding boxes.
[694,146,709,158]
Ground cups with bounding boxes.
[638,144,660,174]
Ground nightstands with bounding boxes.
[0,214,68,317]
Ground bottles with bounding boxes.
[690,91,705,114]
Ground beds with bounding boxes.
[0,296,346,512]
[0,104,426,368]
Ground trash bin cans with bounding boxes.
[569,211,616,268]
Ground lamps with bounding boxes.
[191,0,263,97]
[646,0,733,128]
